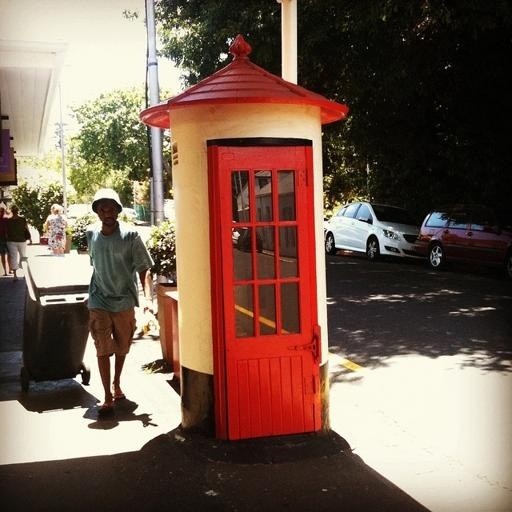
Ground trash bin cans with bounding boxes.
[19,253,93,393]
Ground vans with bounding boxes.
[416,203,512,268]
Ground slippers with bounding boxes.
[98,404,113,412]
[110,393,125,400]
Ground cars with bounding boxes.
[235,226,265,255]
[232,221,249,245]
[162,198,174,225]
[120,206,138,220]
[323,201,433,264]
[66,201,87,218]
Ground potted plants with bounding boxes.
[9,183,63,245]
[70,212,98,254]
[64,227,73,253]
[145,220,177,370]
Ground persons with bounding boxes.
[85,187,156,418]
[42,202,72,255]
[0,207,7,276]
[5,202,34,283]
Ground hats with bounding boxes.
[92,188,123,213]
